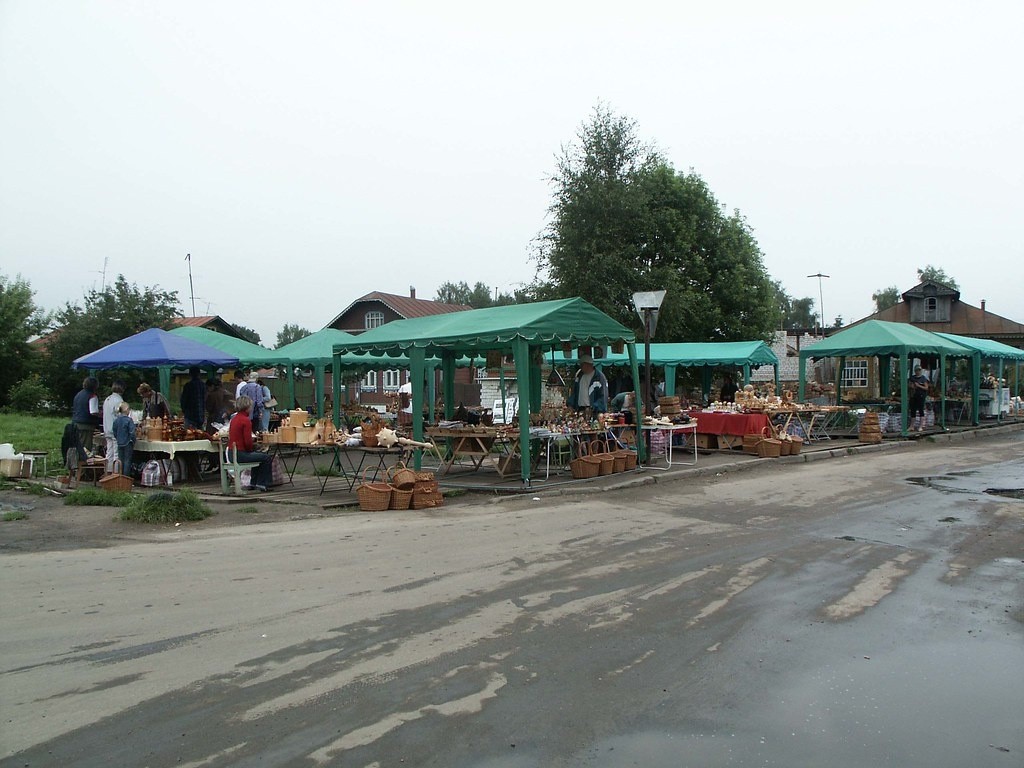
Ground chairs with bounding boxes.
[219,441,260,497]
[61,424,108,483]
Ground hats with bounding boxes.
[915,365,922,370]
[578,355,595,365]
[249,372,259,383]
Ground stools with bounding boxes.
[19,451,48,480]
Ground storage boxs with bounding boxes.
[0,458,31,478]
[497,455,521,474]
[688,433,717,449]
[717,435,743,449]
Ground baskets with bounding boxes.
[98,458,133,494]
[356,461,443,511]
[569,440,637,479]
[754,425,802,458]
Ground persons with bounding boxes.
[981,375,1007,389]
[111,401,139,480]
[103,379,125,477]
[179,366,273,438]
[610,372,762,447]
[564,355,608,471]
[907,365,930,431]
[228,393,273,492]
[397,375,415,413]
[136,383,172,427]
[74,377,103,481]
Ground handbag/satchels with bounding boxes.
[271,455,283,486]
[262,386,279,408]
[141,459,188,486]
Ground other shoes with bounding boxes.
[247,485,256,491]
[918,427,923,432]
[261,488,273,492]
[908,427,914,432]
[66,486,80,492]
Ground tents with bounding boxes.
[333,297,646,483]
[931,330,1024,427]
[541,340,779,397]
[72,326,507,423]
[797,318,980,435]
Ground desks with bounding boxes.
[93,439,219,496]
[268,443,418,496]
[639,422,697,471]
[422,424,638,482]
[770,408,849,447]
[925,399,992,427]
[673,413,771,455]
[837,402,899,434]
